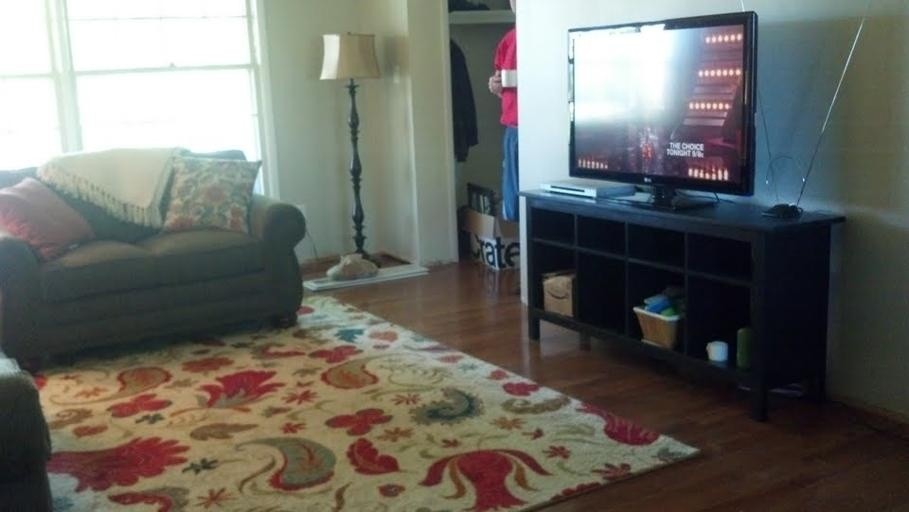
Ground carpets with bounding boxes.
[32,294,704,511]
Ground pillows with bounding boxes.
[0,176,98,265]
[161,154,264,238]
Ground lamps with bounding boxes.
[317,31,383,270]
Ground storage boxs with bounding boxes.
[461,207,519,272]
[540,268,579,319]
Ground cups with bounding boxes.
[501,69,517,89]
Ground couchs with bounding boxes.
[0,356,56,512]
[0,148,308,377]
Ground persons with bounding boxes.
[488,1,518,222]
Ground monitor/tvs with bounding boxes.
[565,11,760,213]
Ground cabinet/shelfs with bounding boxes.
[515,184,847,423]
